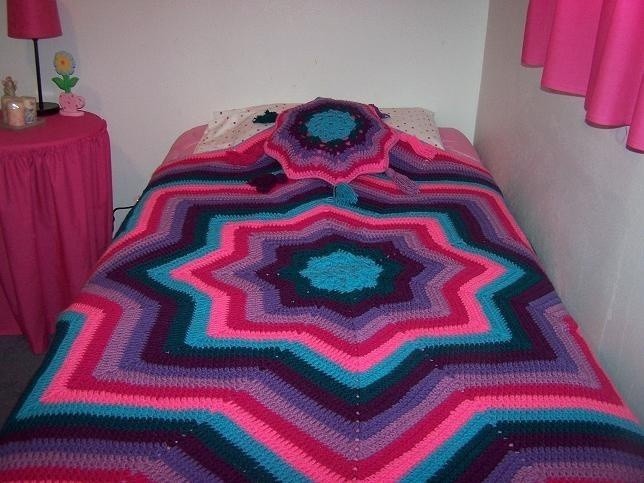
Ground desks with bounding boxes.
[0,110,113,336]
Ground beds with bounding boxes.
[0,125,643,483]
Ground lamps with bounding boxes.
[5,0,62,117]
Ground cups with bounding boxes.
[20,95,39,125]
[2,94,20,125]
[7,99,27,128]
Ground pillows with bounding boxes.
[193,103,444,154]
